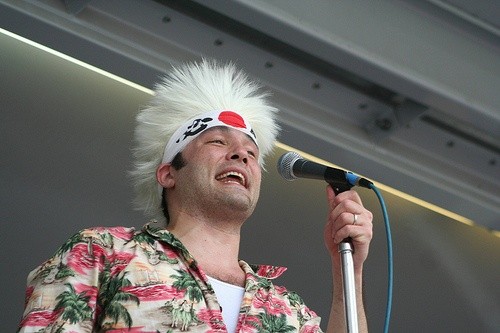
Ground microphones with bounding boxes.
[276,151,374,191]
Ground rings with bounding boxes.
[351,213,358,225]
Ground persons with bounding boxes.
[14,55,374,333]
